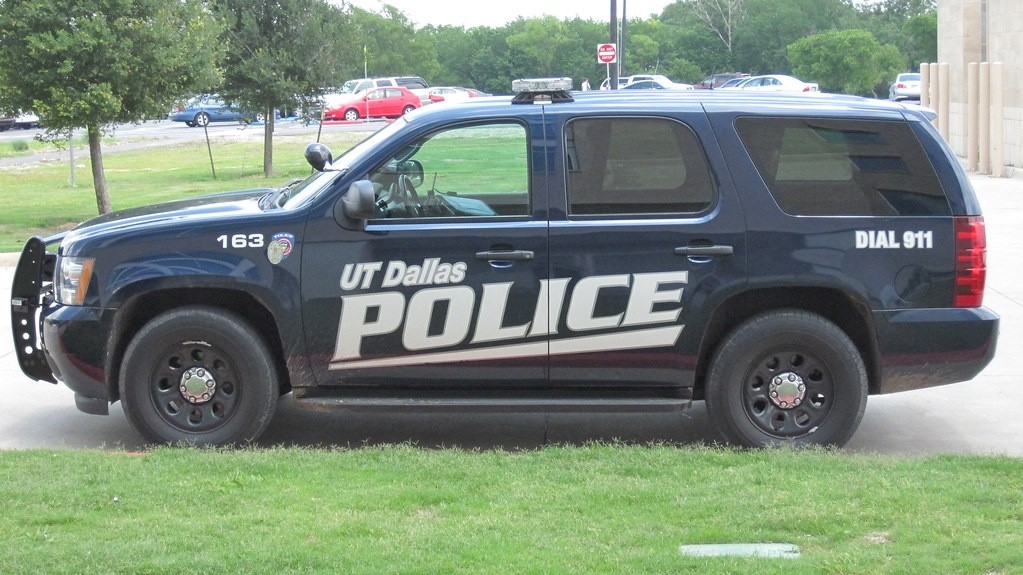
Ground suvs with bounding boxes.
[9,91,998,455]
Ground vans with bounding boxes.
[326,75,428,98]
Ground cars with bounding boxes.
[601,73,693,90]
[419,87,493,105]
[692,72,819,95]
[888,73,920,100]
[168,93,267,127]
[323,86,423,121]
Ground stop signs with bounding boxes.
[599,45,616,62]
